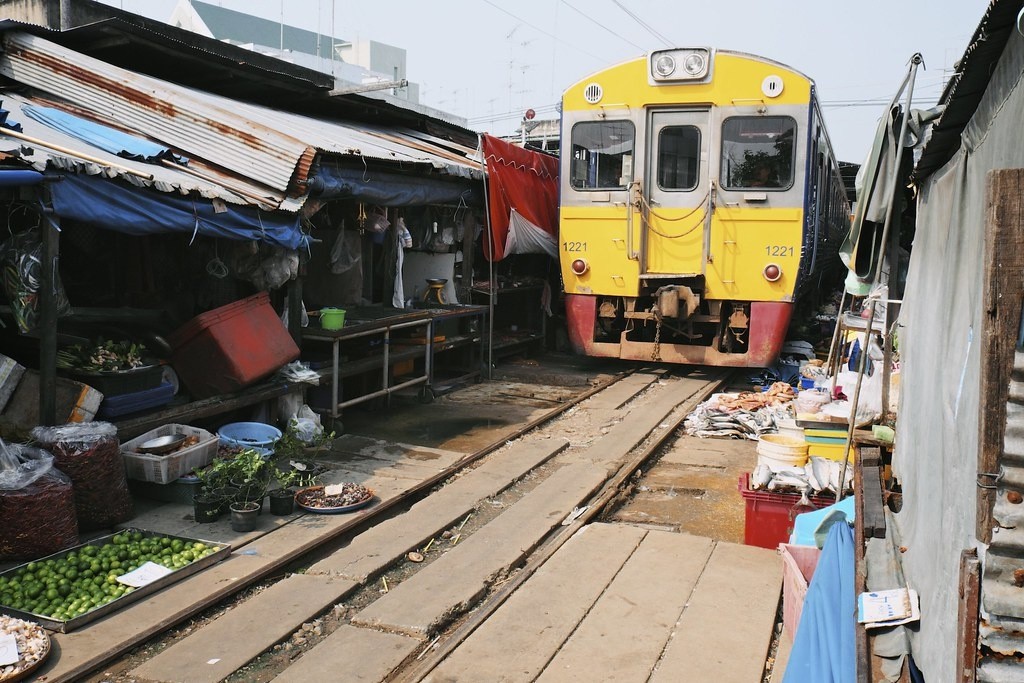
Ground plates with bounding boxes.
[136,433,187,454]
[424,277,448,285]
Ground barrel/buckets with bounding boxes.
[755,433,809,472]
[318,309,347,330]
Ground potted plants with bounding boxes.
[268,418,336,487]
[266,459,323,516]
[190,443,282,532]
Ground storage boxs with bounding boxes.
[0,353,105,425]
[737,473,836,550]
[72,364,164,398]
[804,428,854,463]
[388,344,415,376]
[778,542,822,643]
[799,375,821,391]
[118,423,219,484]
[163,293,301,400]
[146,459,228,504]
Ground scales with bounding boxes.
[421,278,449,308]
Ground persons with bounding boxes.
[750,165,777,187]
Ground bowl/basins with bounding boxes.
[218,421,282,450]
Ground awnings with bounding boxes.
[0,15,561,258]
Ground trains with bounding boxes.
[556,46,852,371]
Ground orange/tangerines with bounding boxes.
[0,531,220,622]
